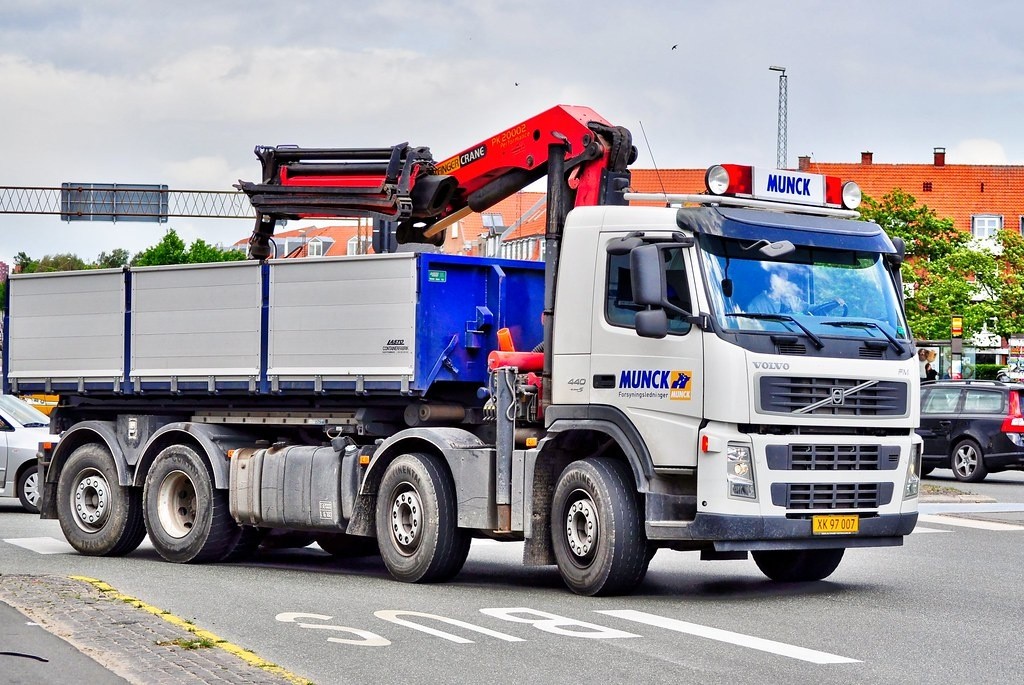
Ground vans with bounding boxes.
[0,389,65,515]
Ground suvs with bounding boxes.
[915,379,1024,484]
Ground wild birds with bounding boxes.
[672,45,677,50]
[515,82,519,86]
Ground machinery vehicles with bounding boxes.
[1,101,925,598]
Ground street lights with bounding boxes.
[768,65,790,171]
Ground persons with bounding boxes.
[924,363,951,379]
[747,266,845,316]
